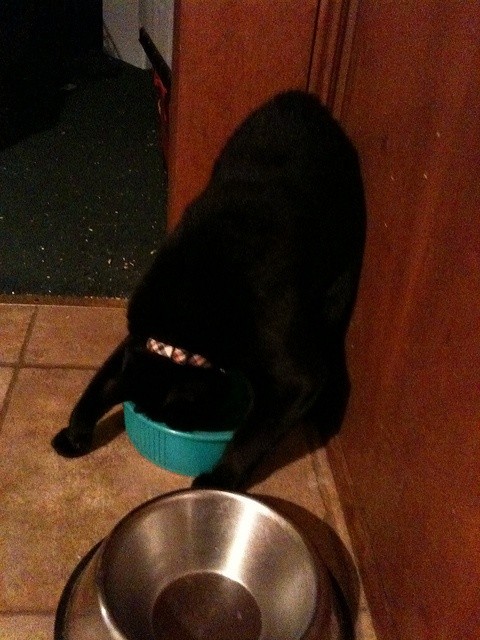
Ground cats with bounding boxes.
[52,89,368,494]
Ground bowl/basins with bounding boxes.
[122,401,234,478]
[95,488,319,640]
